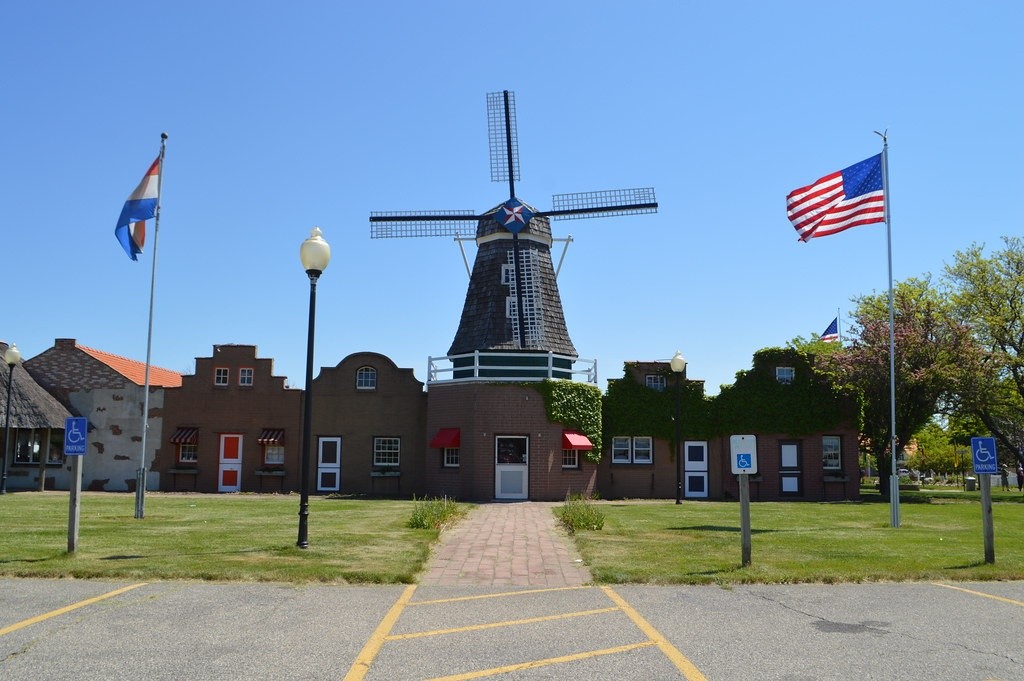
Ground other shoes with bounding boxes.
[1007,489,1010,491]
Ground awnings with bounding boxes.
[562,430,592,450]
[257,429,284,446]
[430,428,461,448]
[169,427,199,445]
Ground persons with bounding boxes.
[1001,464,1012,491]
[1016,464,1024,491]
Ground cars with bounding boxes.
[897,469,910,477]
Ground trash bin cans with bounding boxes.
[965,477,976,491]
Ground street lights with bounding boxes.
[1,343,21,493]
[670,350,687,504]
[295,226,329,547]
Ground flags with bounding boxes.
[820,317,838,342]
[115,145,165,261]
[786,152,886,243]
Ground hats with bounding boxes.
[1003,464,1007,467]
